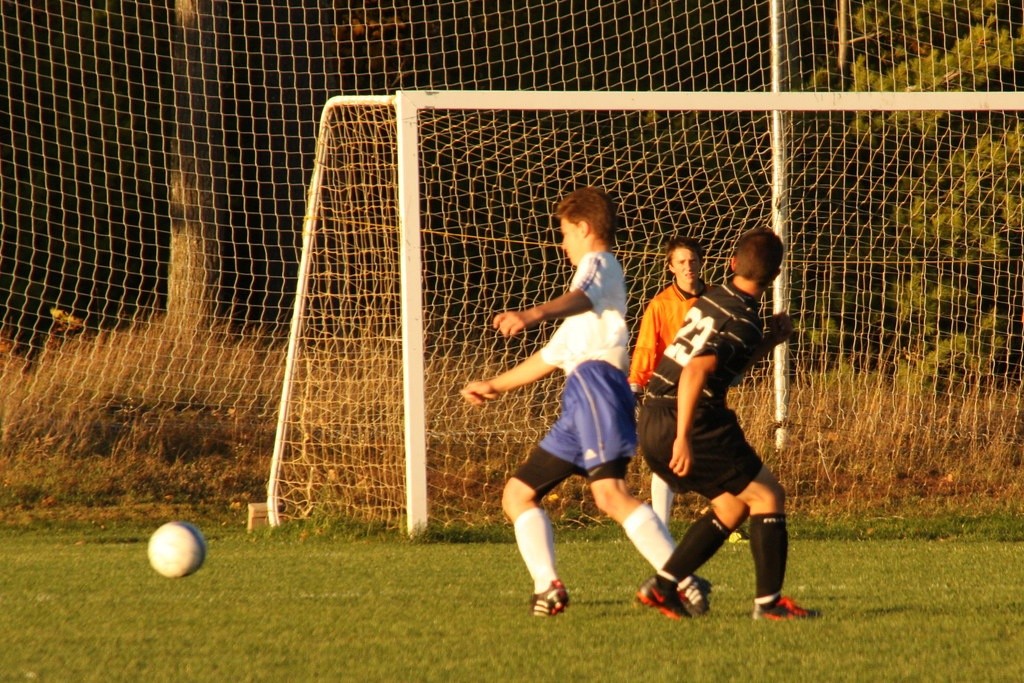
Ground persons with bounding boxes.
[460,189,711,618]
[629,237,750,544]
[634,231,819,621]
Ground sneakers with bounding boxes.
[676,574,712,616]
[637,574,692,622]
[528,578,572,617]
[752,594,822,622]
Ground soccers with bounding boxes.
[143,519,208,580]
